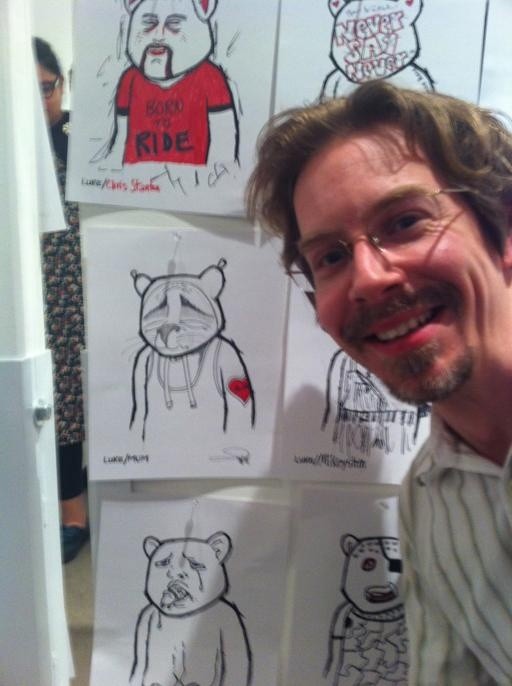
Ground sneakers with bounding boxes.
[59,518,93,566]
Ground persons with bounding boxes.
[30,34,89,561]
[242,75,510,682]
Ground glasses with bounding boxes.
[39,73,64,101]
[292,181,482,284]
